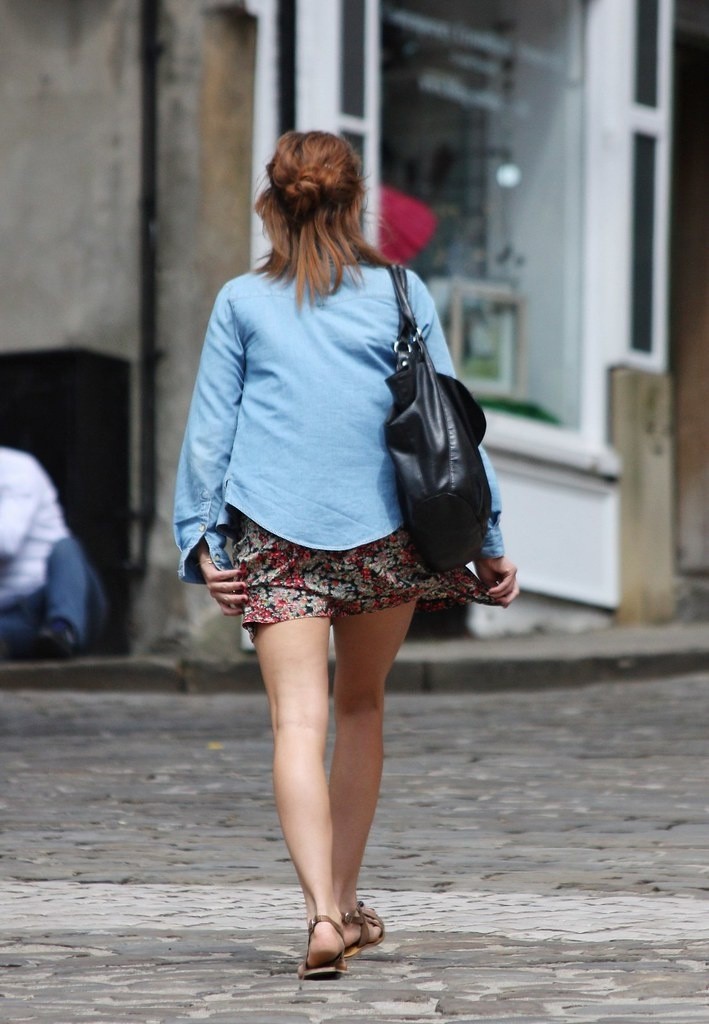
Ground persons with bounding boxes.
[0,447,105,663]
[170,129,520,978]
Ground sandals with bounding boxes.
[296,913,348,976]
[338,900,385,960]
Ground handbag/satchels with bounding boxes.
[383,261,493,575]
